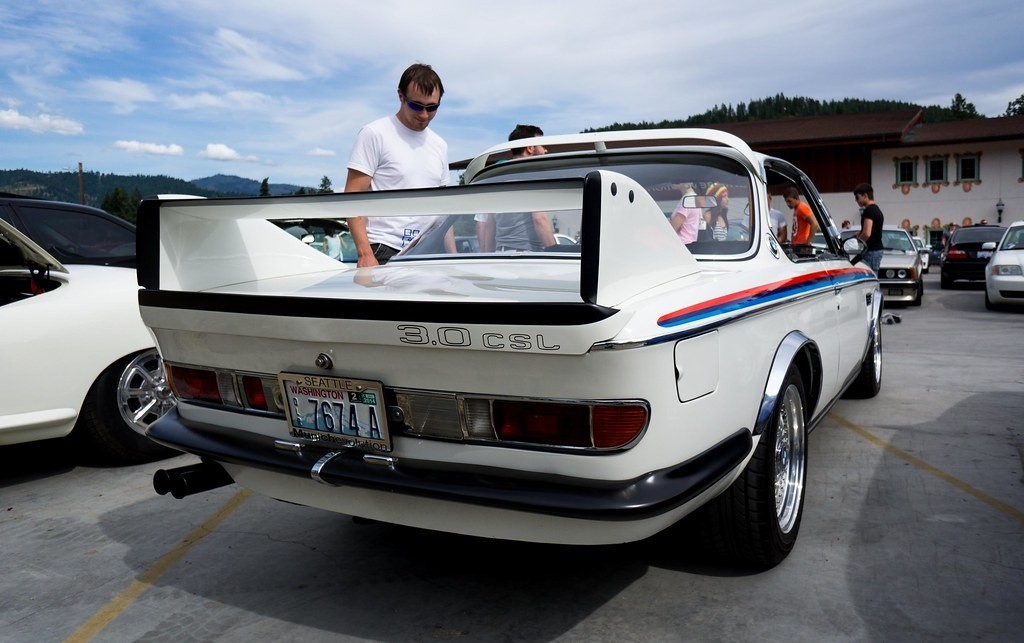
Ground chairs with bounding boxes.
[1019,233,1024,243]
[887,239,903,250]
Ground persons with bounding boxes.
[942,221,956,248]
[352,266,458,300]
[839,221,851,239]
[476,122,555,252]
[343,61,460,270]
[851,182,884,279]
[981,219,987,226]
[663,176,821,259]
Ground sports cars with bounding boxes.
[138,121,884,576]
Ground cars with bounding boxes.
[899,235,933,273]
[982,221,1024,310]
[0,216,191,465]
[812,232,832,256]
[838,225,932,306]
[552,233,581,245]
[0,197,141,269]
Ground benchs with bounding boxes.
[542,241,751,255]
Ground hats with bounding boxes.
[705,182,728,201]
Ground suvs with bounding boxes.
[935,222,1018,290]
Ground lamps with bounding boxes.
[552,215,558,228]
[996,199,1005,223]
[859,206,865,214]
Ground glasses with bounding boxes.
[402,96,440,112]
[855,194,862,200]
